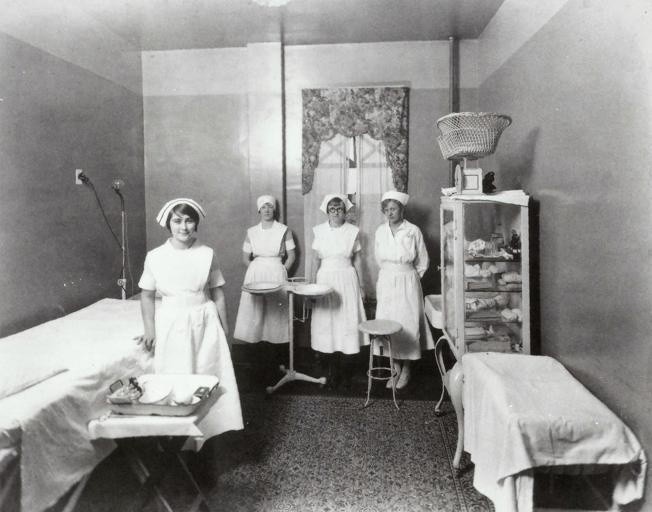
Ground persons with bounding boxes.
[132,197,245,488]
[233,195,297,391]
[309,192,372,389]
[374,190,431,391]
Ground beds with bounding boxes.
[448,353,648,511]
[0,293,162,511]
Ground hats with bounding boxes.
[380,191,409,206]
[256,195,277,213]
[319,194,354,216]
[156,198,206,227]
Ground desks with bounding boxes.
[90,387,226,511]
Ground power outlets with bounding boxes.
[75,168,83,185]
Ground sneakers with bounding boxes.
[396,372,411,389]
[385,370,401,389]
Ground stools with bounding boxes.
[356,318,403,416]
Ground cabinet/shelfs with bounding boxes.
[438,190,530,354]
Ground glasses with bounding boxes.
[328,207,343,213]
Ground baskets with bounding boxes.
[437,111,512,161]
